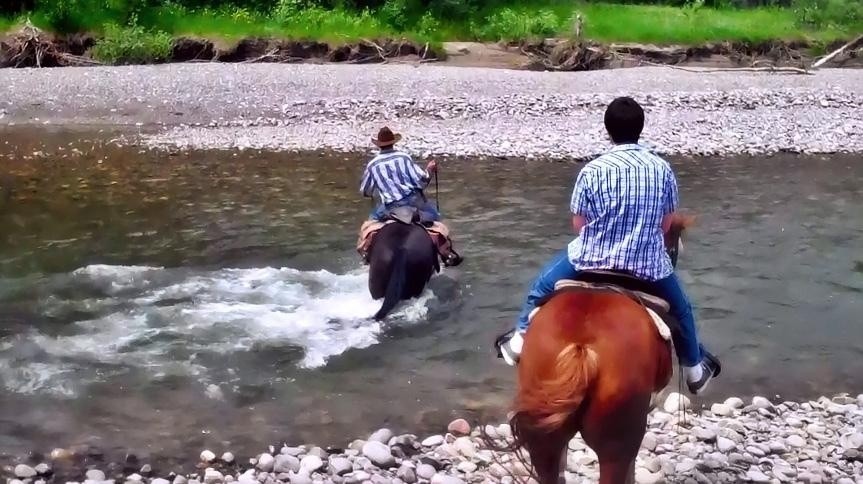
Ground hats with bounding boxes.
[372,126,402,147]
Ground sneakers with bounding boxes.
[686,354,721,395]
[497,335,522,366]
[441,252,464,267]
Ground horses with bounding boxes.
[509,283,675,484]
[365,220,440,323]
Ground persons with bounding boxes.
[359,124,458,268]
[493,94,720,396]
